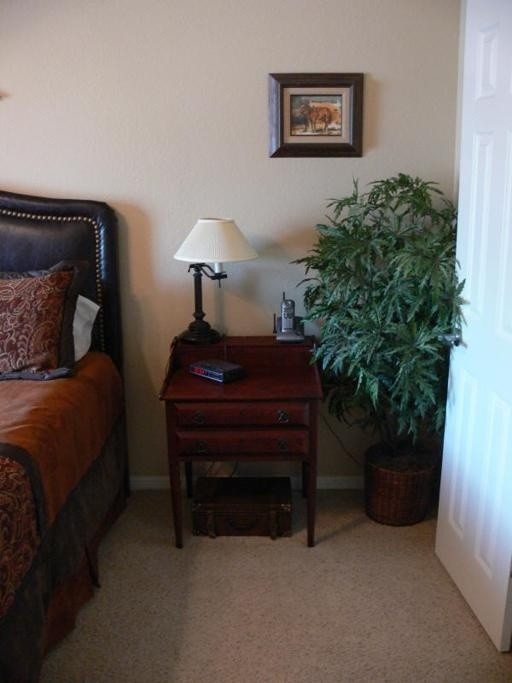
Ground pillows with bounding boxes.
[1,258,84,380]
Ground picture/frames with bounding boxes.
[267,72,364,158]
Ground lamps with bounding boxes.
[173,216,255,345]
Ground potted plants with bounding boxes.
[294,174,465,527]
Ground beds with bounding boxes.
[0,184,133,677]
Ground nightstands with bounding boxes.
[158,336,323,550]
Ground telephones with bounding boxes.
[273,291,306,343]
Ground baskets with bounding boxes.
[364,441,434,526]
